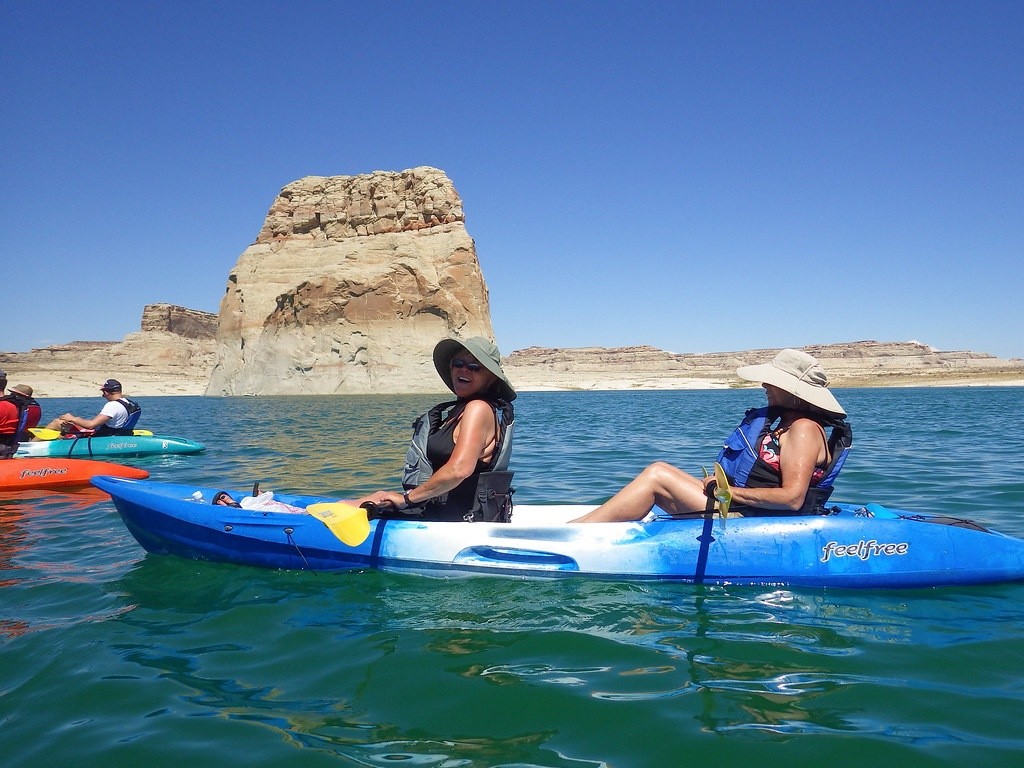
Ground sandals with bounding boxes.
[253,482,265,497]
[213,491,242,508]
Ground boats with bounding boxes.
[0,457,150,491]
[91,472,1024,588]
[13,434,207,457]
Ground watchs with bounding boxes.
[403,488,419,507]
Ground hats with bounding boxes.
[7,384,33,398]
[433,336,517,403]
[736,349,848,419]
[99,379,121,390]
[0,367,7,379]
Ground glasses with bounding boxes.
[452,359,483,371]
[102,394,105,397]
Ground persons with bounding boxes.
[567,348,848,523]
[30,378,142,442]
[211,336,517,521]
[0,367,42,460]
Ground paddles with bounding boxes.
[305,500,395,548]
[700,461,733,520]
[27,427,154,440]
